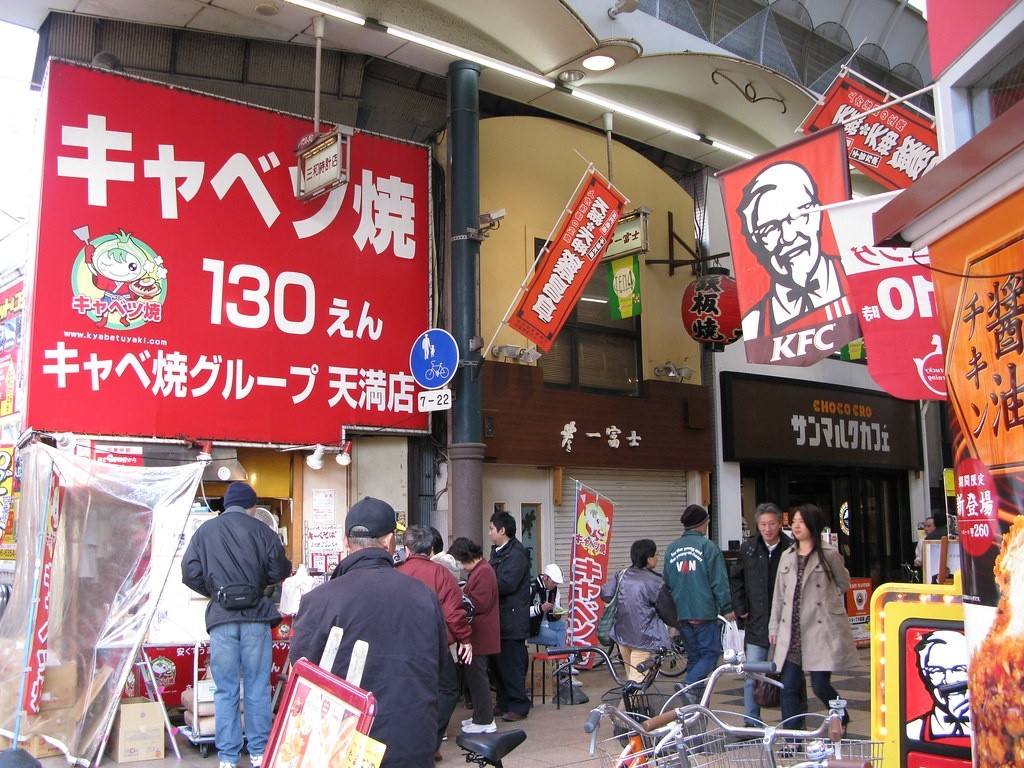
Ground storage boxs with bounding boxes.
[0,661,113,758]
[181,680,276,736]
[105,696,164,763]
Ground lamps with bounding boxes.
[335,442,353,465]
[492,344,541,362]
[654,362,695,378]
[306,445,326,469]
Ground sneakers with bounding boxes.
[558,675,583,687]
[461,718,474,726]
[461,720,497,734]
[558,663,579,675]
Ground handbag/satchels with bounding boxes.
[596,567,628,647]
[722,618,745,663]
[753,638,781,710]
[210,583,264,609]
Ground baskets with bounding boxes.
[601,681,885,768]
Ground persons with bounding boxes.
[393,525,500,733]
[663,504,734,724]
[769,504,861,758]
[531,564,583,687]
[182,482,285,768]
[600,539,681,725]
[488,512,531,720]
[289,497,447,768]
[914,511,947,583]
[719,503,795,745]
[719,626,729,627]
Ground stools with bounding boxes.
[530,653,574,709]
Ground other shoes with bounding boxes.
[501,710,527,721]
[831,720,849,745]
[735,723,760,743]
[778,744,802,758]
[492,705,505,716]
[249,753,263,767]
[674,681,696,708]
[435,750,443,760]
[465,695,473,710]
[219,761,237,768]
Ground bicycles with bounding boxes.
[584,648,785,768]
[647,696,885,768]
[456,645,672,768]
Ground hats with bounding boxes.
[680,504,709,529]
[345,496,397,538]
[544,564,563,583]
[223,482,257,510]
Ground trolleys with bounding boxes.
[177,639,249,759]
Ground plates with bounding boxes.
[548,610,568,615]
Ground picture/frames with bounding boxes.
[257,660,378,768]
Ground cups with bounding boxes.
[782,511,788,527]
